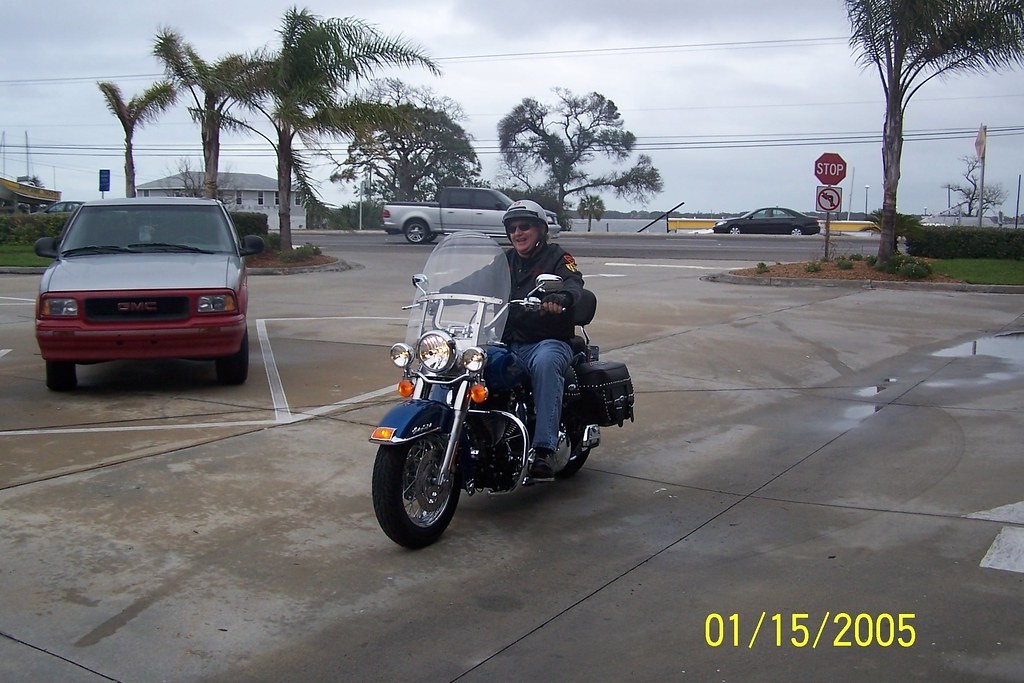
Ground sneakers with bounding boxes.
[530,447,556,478]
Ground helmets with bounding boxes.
[501,199,549,233]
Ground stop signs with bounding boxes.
[814,152,847,185]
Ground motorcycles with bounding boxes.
[367,231,636,551]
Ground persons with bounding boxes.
[416,200,584,482]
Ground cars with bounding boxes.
[34,196,266,392]
[712,207,820,235]
[30,200,86,215]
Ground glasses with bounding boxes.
[507,224,531,233]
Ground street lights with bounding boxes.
[865,185,870,219]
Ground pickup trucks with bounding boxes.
[383,187,562,245]
